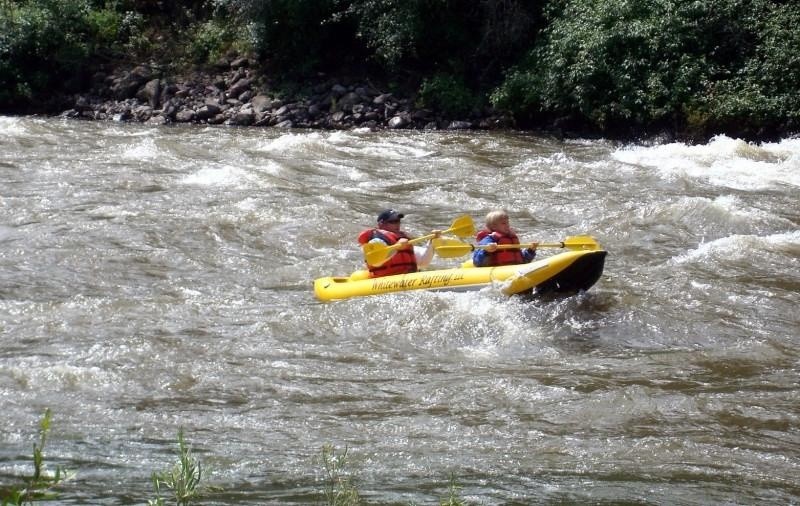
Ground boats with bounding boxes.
[312,248,606,304]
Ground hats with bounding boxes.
[378,209,403,224]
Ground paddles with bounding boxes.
[363,215,474,268]
[431,235,598,259]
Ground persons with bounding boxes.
[472,210,539,267]
[359,208,442,278]
